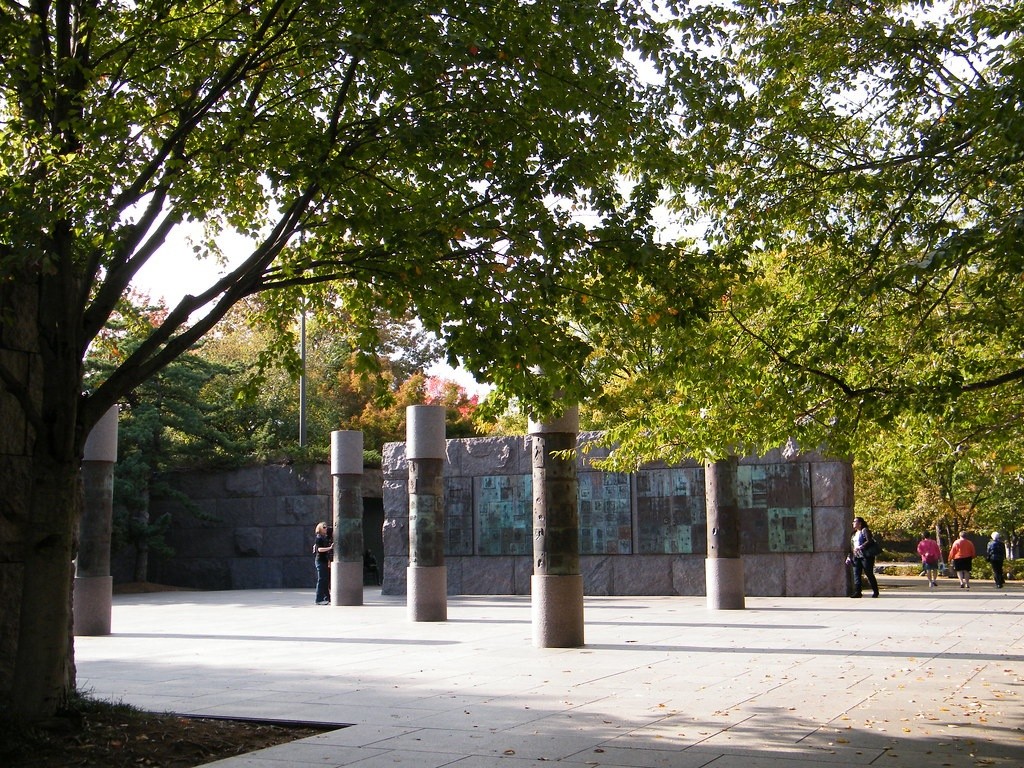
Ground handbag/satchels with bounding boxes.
[860,528,884,558]
[986,548,994,562]
[926,554,936,564]
[312,544,316,558]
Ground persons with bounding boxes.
[986,531,1006,588]
[947,530,976,591]
[845,516,879,598]
[363,548,382,586]
[314,522,334,605]
[917,530,941,587]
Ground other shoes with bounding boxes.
[315,600,329,605]
[961,584,964,588]
[966,586,970,588]
[998,584,1003,588]
[932,582,937,586]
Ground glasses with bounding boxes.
[321,527,327,529]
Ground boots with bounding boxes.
[872,583,879,598]
[850,583,862,598]
